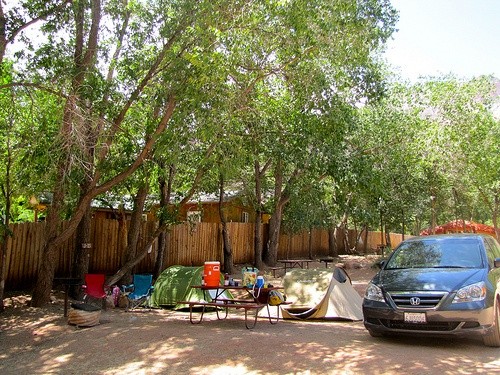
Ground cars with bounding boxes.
[363,234,500,348]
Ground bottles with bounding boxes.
[224,273,230,286]
[256,276,264,288]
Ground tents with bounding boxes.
[141,264,239,310]
[253,266,363,321]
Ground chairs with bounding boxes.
[82,274,107,311]
[122,274,155,312]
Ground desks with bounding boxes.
[189,285,286,329]
[278,260,312,273]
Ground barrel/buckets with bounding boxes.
[203,261,220,287]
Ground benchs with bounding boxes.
[270,266,298,278]
[212,298,292,325]
[177,302,263,329]
